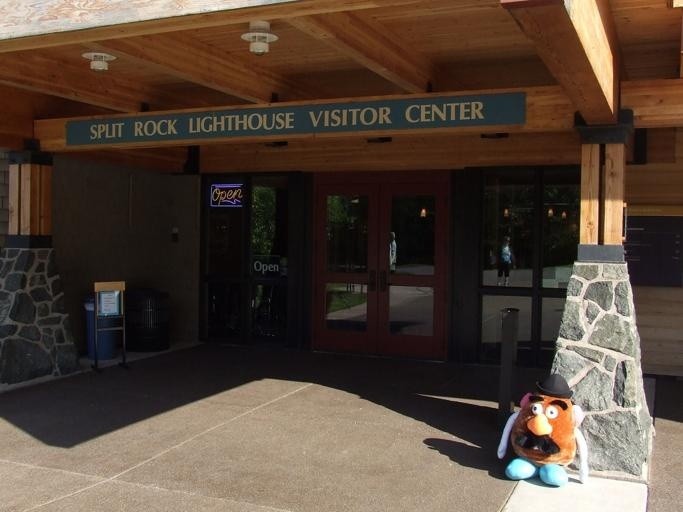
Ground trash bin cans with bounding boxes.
[82,292,119,360]
[126,288,170,352]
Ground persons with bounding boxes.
[389,230,398,271]
[497,236,516,287]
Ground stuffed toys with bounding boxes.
[497,374,590,487]
